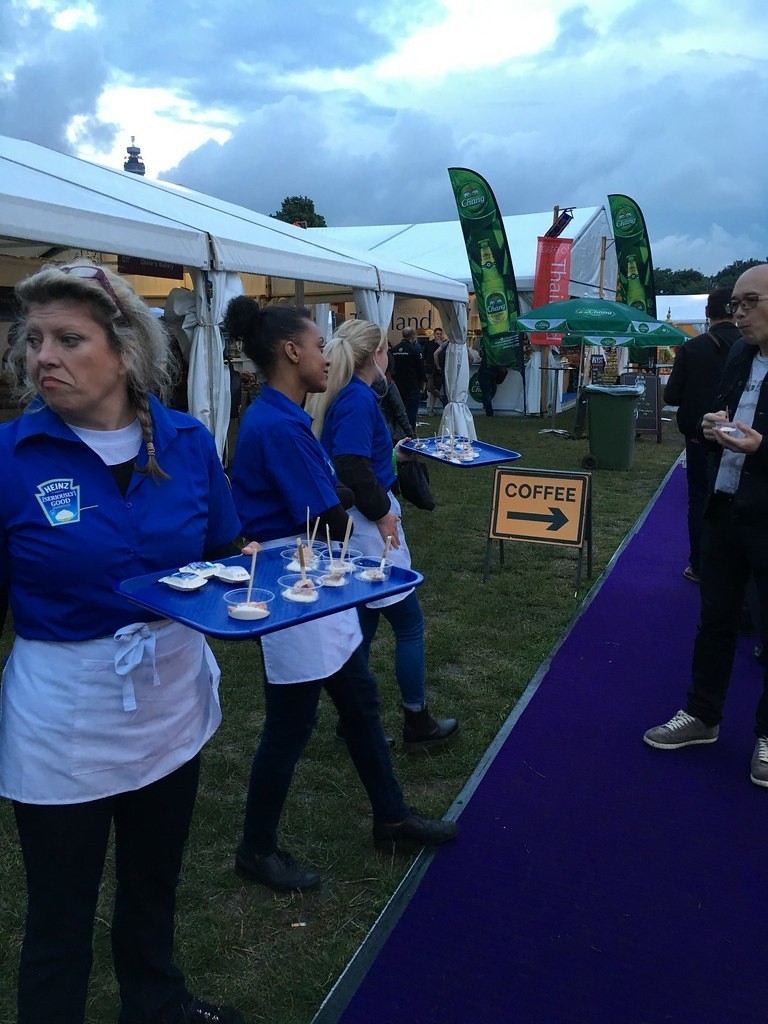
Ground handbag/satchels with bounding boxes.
[399,459,436,511]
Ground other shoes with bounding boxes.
[683,567,701,581]
[121,1001,244,1024]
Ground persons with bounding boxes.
[389,326,424,432]
[434,341,474,371]
[642,263,768,789]
[423,328,444,417]
[218,297,458,894]
[663,288,742,585]
[737,592,767,668]
[0,257,264,1024]
[304,318,459,754]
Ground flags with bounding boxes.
[447,168,527,385]
[608,195,657,375]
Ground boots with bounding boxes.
[400,705,459,746]
[337,717,397,750]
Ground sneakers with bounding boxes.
[750,736,768,787]
[235,846,321,892]
[643,710,720,749]
[374,807,458,849]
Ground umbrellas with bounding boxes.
[508,296,694,385]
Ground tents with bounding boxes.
[0,136,477,443]
[304,204,621,415]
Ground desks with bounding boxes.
[539,366,577,435]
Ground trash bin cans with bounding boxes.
[581,384,645,471]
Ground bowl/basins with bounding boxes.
[277,573,325,603]
[411,435,481,464]
[715,421,736,432]
[280,539,394,587]
[223,587,275,621]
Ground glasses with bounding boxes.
[33,266,135,327]
[725,295,768,314]
